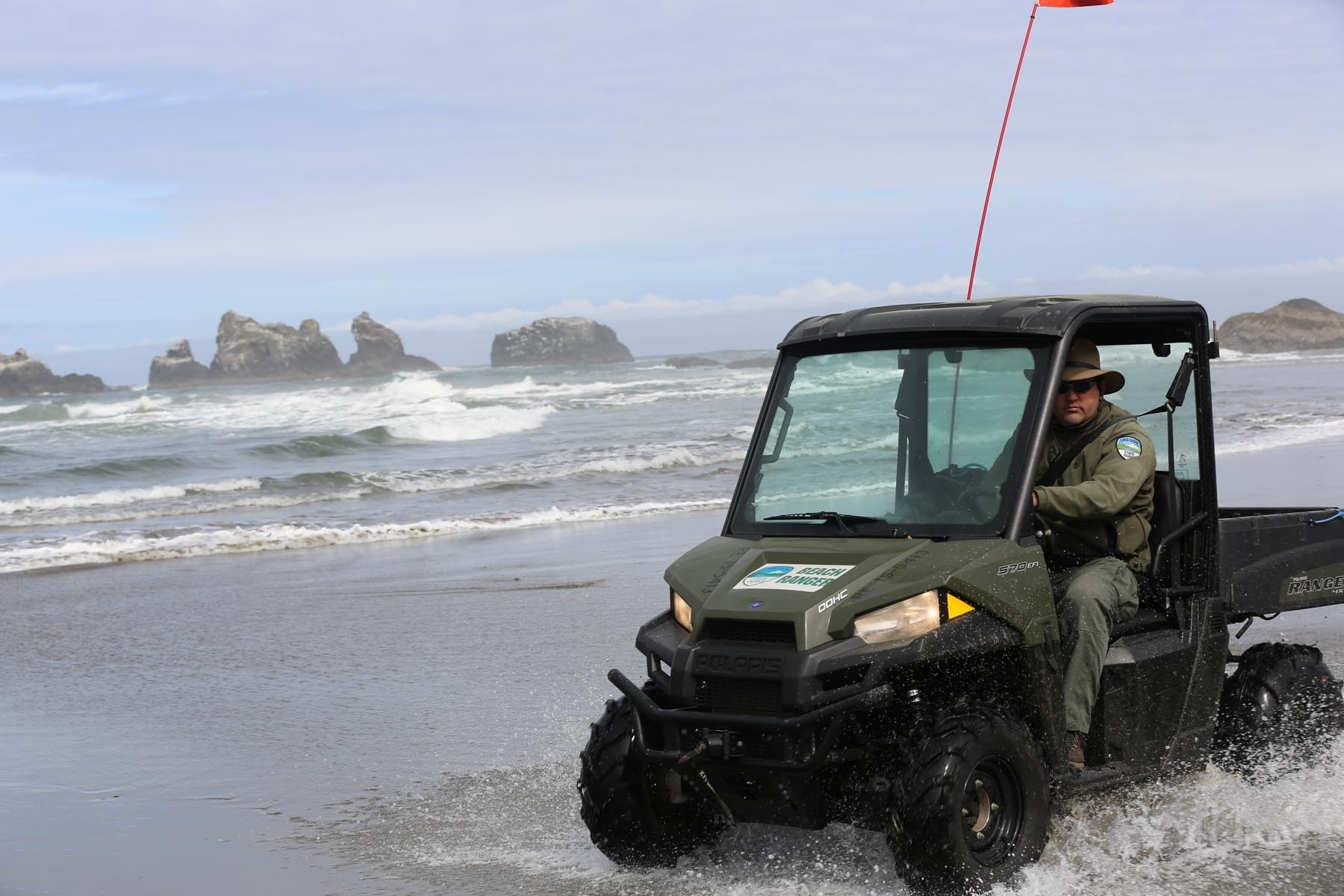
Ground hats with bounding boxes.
[1024,344,1125,396]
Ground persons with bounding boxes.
[976,345,1157,771]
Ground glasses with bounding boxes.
[1058,378,1098,395]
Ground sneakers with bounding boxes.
[1065,731,1087,771]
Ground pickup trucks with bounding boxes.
[577,291,1344,896]
